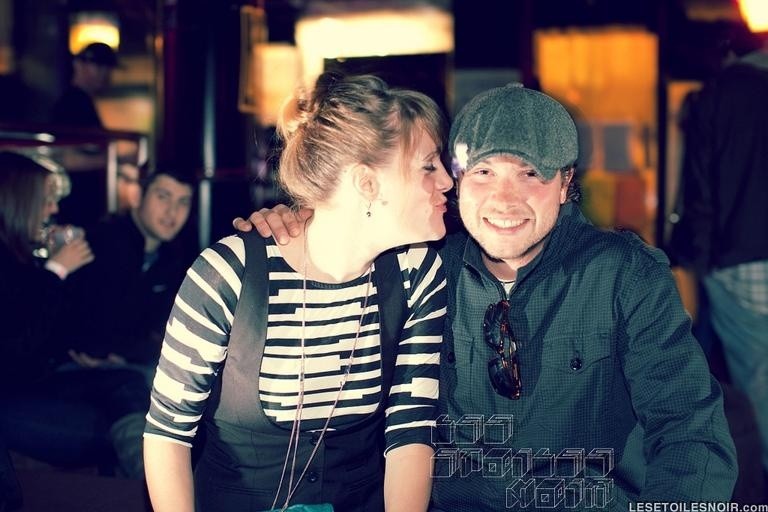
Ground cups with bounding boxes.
[54,227,84,254]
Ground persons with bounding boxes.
[228,79,742,510]
[138,65,458,511]
[40,39,129,220]
[41,154,204,477]
[0,148,99,463]
[661,1,767,469]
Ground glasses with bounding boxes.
[153,187,193,208]
[484,300,521,400]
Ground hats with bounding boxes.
[449,82,579,181]
[77,42,125,70]
